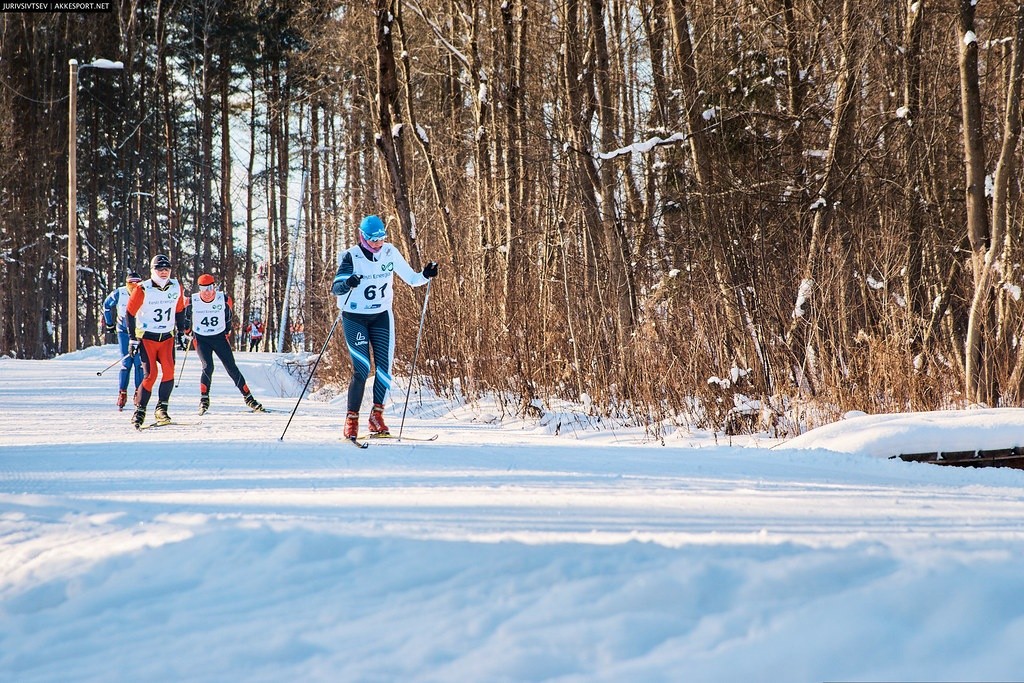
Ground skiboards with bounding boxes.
[123,421,203,429]
[340,432,439,449]
[198,409,294,416]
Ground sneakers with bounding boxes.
[131,406,146,431]
[155,403,172,426]
[366,411,391,439]
[343,411,359,440]
[117,389,127,411]
[244,395,265,412]
[199,397,210,414]
[133,391,139,404]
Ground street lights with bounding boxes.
[68,59,124,353]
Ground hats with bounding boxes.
[361,215,386,241]
[198,274,215,286]
[126,272,142,282]
[151,255,172,267]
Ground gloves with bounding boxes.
[423,260,438,278]
[346,274,361,288]
[177,332,192,349]
[106,326,114,335]
[128,339,139,358]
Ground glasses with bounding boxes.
[199,285,213,290]
[156,267,170,271]
[370,238,385,242]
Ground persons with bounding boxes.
[330,215,438,440]
[184,274,270,416]
[126,255,185,429]
[102,273,144,412]
[244,318,265,352]
[176,328,193,351]
